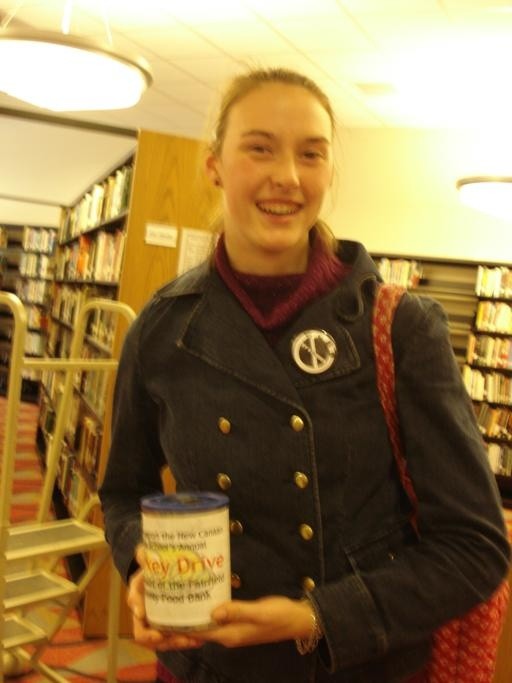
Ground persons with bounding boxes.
[95,65,511,683]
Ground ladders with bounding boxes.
[0,292,137,683]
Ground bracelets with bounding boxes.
[295,596,322,655]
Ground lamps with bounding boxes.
[1,29,155,113]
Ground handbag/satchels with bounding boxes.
[372,281,507,683]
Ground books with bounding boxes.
[366,248,511,478]
[1,150,136,526]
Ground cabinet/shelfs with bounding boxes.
[0,129,512,639]
